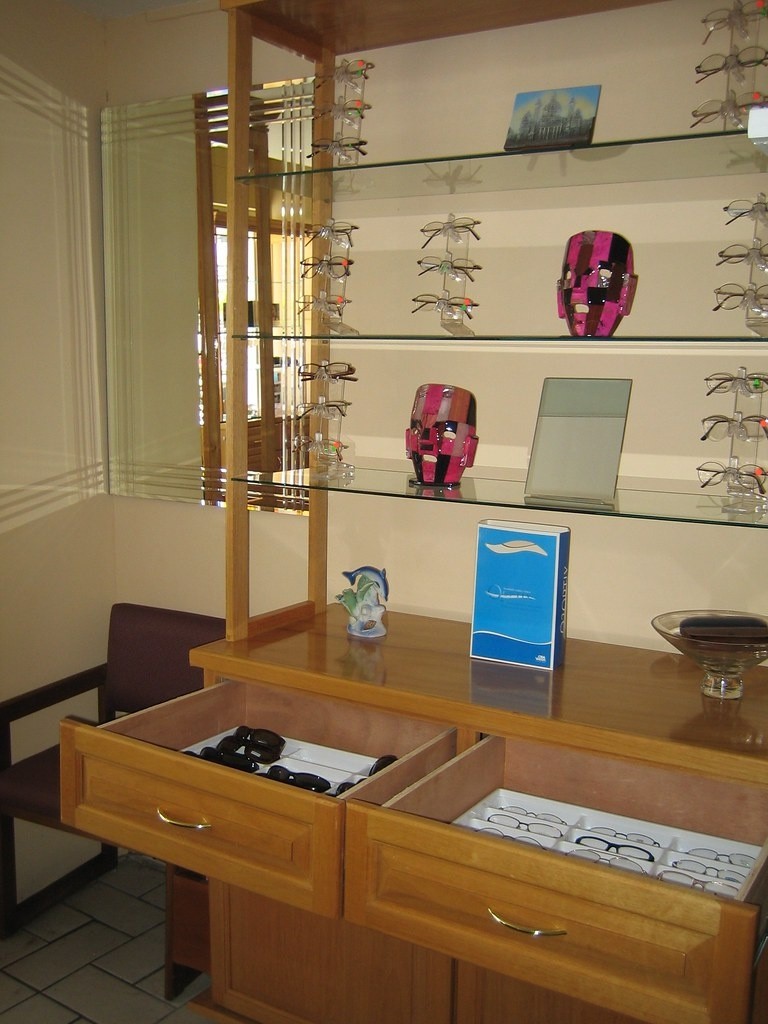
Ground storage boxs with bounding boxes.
[470,519,572,671]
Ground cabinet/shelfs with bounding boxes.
[53,0,768,1024]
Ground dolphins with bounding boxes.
[341,565,390,603]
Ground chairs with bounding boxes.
[0,601,227,1001]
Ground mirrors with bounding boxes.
[96,73,311,517]
[0,53,110,527]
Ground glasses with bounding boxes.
[419,213,482,249]
[311,95,373,121]
[306,137,369,158]
[294,399,353,420]
[469,805,757,899]
[303,217,359,248]
[689,0,768,489]
[295,289,353,316]
[416,255,483,282]
[314,58,375,89]
[292,433,349,462]
[181,725,398,796]
[298,359,359,383]
[300,255,354,279]
[411,293,480,320]
